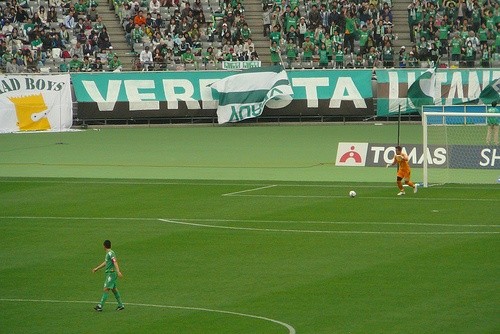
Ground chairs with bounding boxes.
[268,0,361,68]
[120,0,235,71]
[412,26,500,68]
[0,0,110,72]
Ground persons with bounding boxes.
[93,239,126,311]
[0,0,500,69]
[386,146,418,195]
[486,101,500,148]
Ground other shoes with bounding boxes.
[397,191,406,196]
[413,184,417,194]
[93,304,103,312]
[115,305,125,311]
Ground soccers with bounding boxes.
[350,191,356,197]
